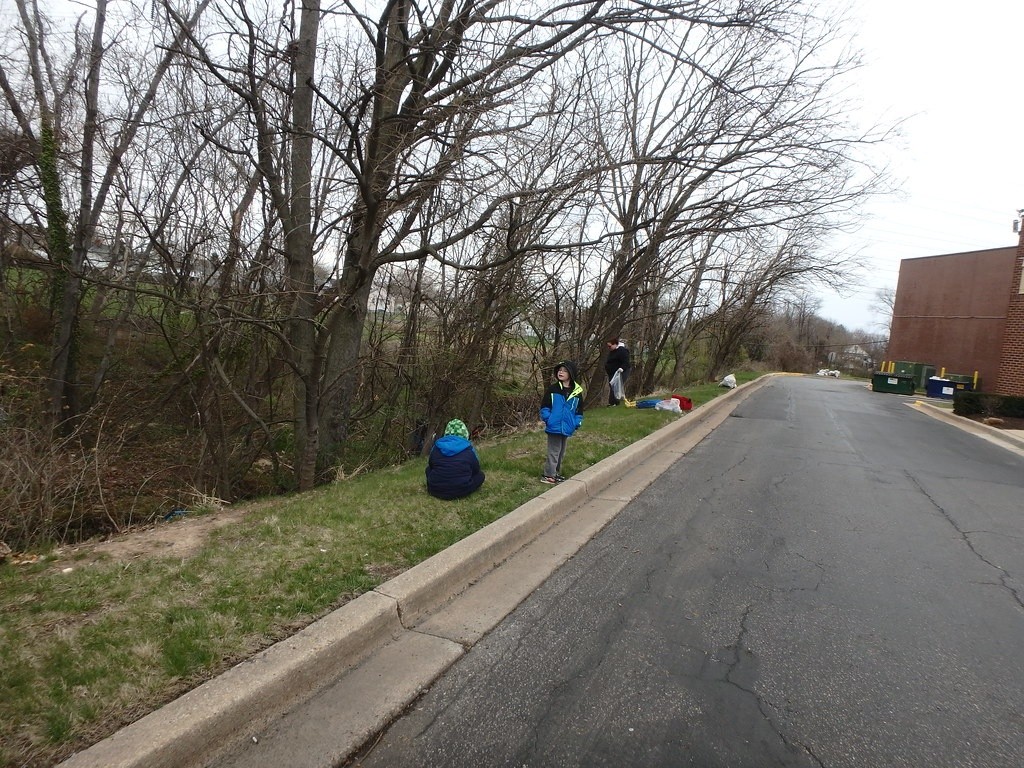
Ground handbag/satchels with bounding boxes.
[609,371,625,399]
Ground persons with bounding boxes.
[539,360,584,484]
[604,338,630,407]
[425,418,486,500]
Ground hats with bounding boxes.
[445,419,468,439]
[553,361,578,380]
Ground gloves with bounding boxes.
[618,368,623,372]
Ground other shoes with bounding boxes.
[541,476,559,484]
[556,474,565,482]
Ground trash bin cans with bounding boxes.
[870,371,917,396]
[927,379,971,400]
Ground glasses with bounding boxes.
[609,345,612,347]
